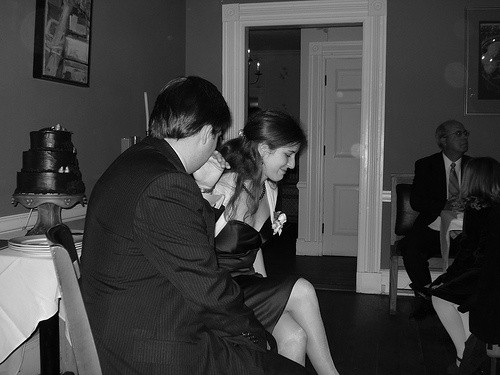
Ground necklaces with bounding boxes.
[241,181,266,200]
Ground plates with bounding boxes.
[7,235,82,254]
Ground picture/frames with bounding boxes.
[30,0,95,90]
[463,4,500,118]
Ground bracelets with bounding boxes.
[194,178,215,193]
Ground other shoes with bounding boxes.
[413,297,426,320]
[451,336,487,375]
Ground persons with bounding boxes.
[80,75,272,375]
[204,108,341,375]
[398,119,474,318]
[408,156,500,375]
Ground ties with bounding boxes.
[448,162,459,204]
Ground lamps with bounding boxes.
[247,47,264,85]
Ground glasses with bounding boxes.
[444,130,470,139]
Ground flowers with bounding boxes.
[271,210,287,239]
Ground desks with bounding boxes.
[0,215,106,375]
[440,206,464,279]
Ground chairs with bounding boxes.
[45,222,97,375]
[390,171,455,317]
